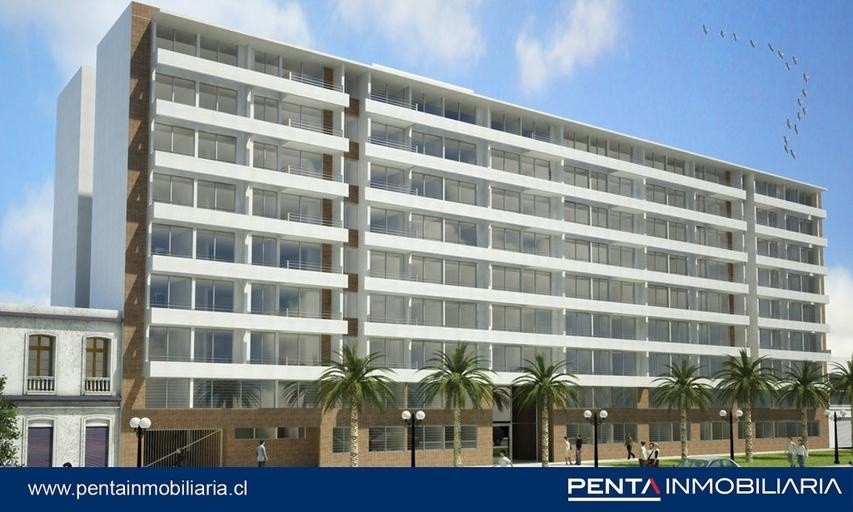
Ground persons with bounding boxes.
[784,436,796,467]
[794,439,809,467]
[63,462,71,467]
[574,432,583,464]
[563,436,572,465]
[174,448,184,467]
[256,439,268,466]
[624,434,659,466]
[499,451,514,466]
